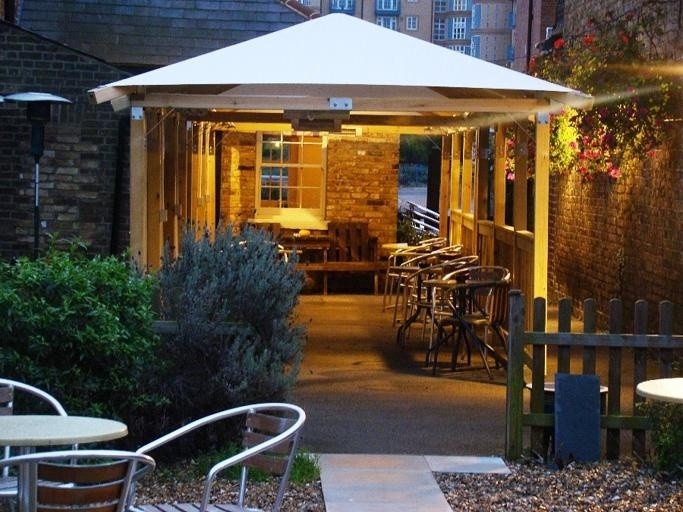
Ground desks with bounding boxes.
[1,415,129,511]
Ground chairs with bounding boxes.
[0,450,158,512]
[249,222,380,297]
[0,378,77,512]
[128,402,306,512]
[381,236,514,381]
